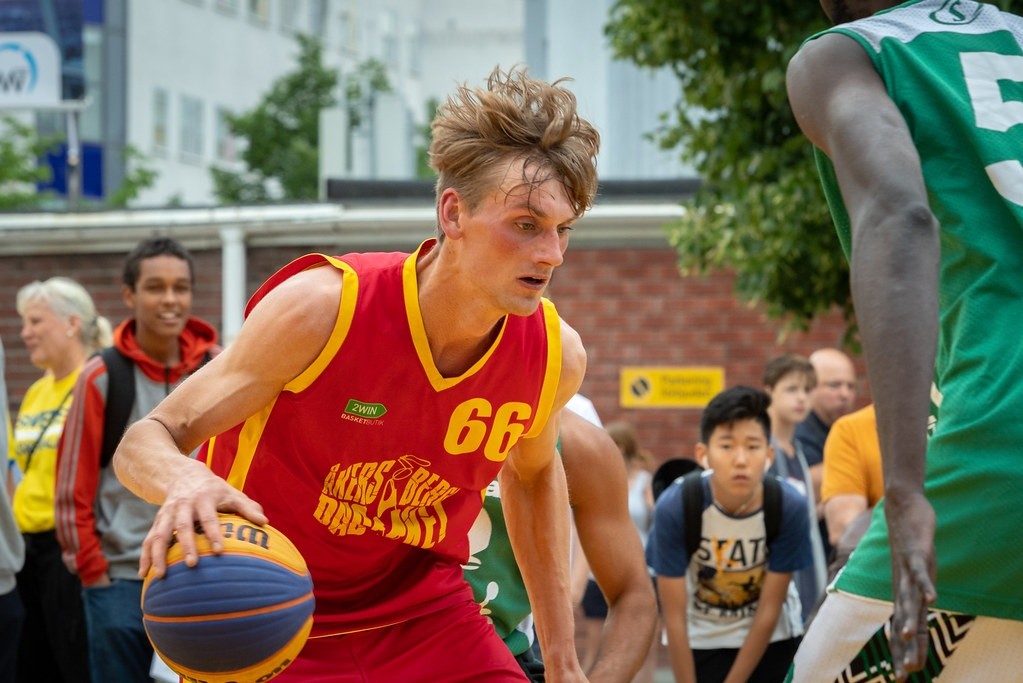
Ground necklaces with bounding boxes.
[711,485,758,517]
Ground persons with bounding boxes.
[0,0,1023,683]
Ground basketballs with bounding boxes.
[138,510,319,683]
